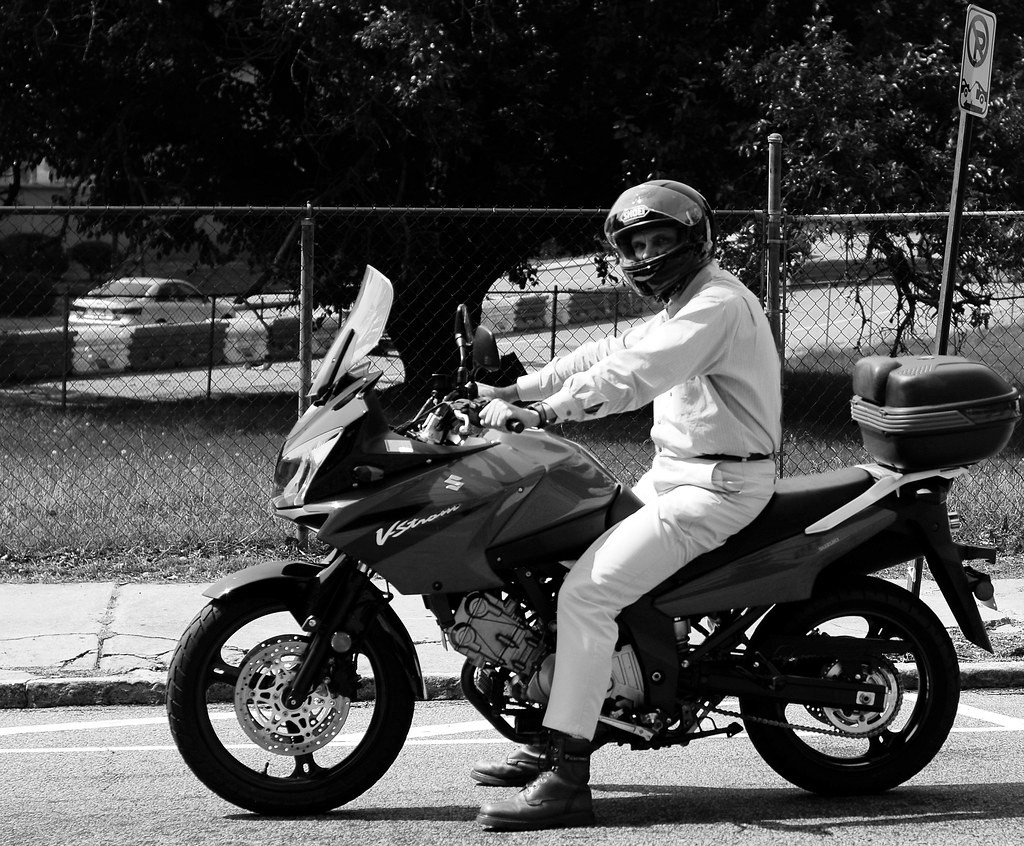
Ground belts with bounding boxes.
[692,453,769,462]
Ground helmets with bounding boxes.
[604,180,716,298]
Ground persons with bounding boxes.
[465,181,780,826]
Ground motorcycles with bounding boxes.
[165,264,1020,817]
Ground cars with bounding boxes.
[67,277,235,328]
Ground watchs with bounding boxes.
[527,402,547,429]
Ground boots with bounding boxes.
[476,729,594,830]
[471,742,548,786]
